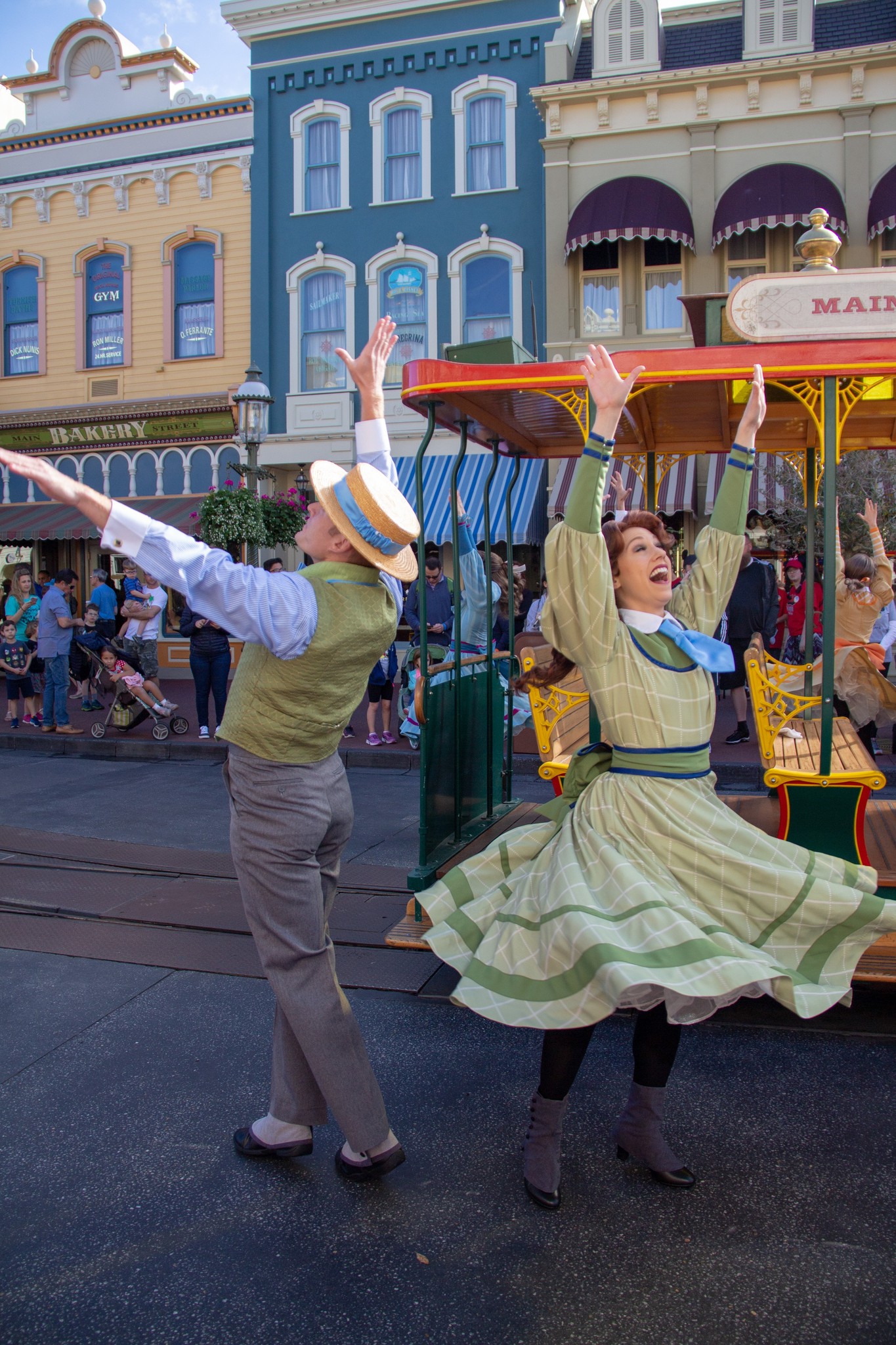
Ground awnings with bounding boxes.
[0,496,210,541]
[712,164,849,253]
[393,455,548,546]
[547,459,698,521]
[817,454,896,504]
[563,176,696,266]
[705,453,804,515]
[866,165,896,243]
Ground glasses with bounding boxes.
[65,582,75,590]
[124,570,137,574]
[90,575,95,578]
[425,569,442,578]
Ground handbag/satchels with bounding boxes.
[109,703,135,726]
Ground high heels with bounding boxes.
[617,1142,696,1186]
[525,1175,561,1210]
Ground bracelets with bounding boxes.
[21,607,25,611]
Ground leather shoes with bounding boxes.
[335,1142,406,1180]
[233,1125,314,1160]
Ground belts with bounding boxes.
[98,619,115,623]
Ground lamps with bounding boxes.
[294,463,309,496]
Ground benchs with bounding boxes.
[521,643,614,797]
[744,632,886,866]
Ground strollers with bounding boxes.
[72,631,189,740]
[397,630,451,750]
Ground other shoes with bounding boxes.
[4,692,106,734]
[163,699,179,711]
[744,687,751,700]
[403,706,411,714]
[156,706,172,718]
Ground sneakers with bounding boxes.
[114,634,124,649]
[871,738,883,754]
[366,733,382,746]
[198,726,210,739]
[214,726,220,738]
[132,632,145,647]
[382,730,397,744]
[725,729,750,743]
[342,726,355,738]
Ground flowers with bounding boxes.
[190,480,311,552]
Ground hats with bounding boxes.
[783,559,801,570]
[309,459,422,583]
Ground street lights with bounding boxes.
[232,358,275,572]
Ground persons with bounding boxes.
[408,343,896,1212]
[711,533,823,744]
[263,557,286,573]
[868,599,896,755]
[179,605,230,738]
[766,497,896,762]
[0,315,424,1181]
[399,484,533,742]
[492,561,549,681]
[0,558,168,733]
[343,557,463,746]
[672,549,697,589]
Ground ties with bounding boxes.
[659,620,736,673]
